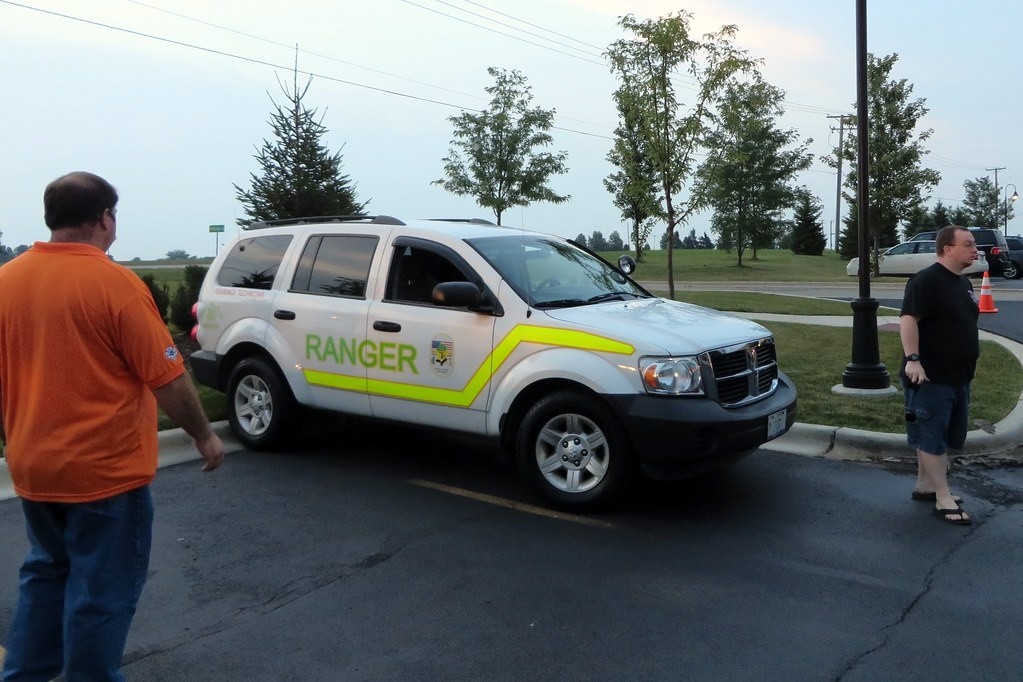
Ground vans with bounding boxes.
[908,229,1012,274]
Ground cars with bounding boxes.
[846,241,990,277]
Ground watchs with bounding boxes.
[906,354,919,361]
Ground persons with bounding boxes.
[0,171,224,682]
[899,226,980,525]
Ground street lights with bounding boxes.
[1005,184,1019,237]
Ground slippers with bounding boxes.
[931,504,973,527]
[912,487,963,505]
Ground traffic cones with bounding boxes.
[976,272,998,313]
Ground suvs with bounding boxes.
[189,215,799,509]
[998,236,1023,280]
